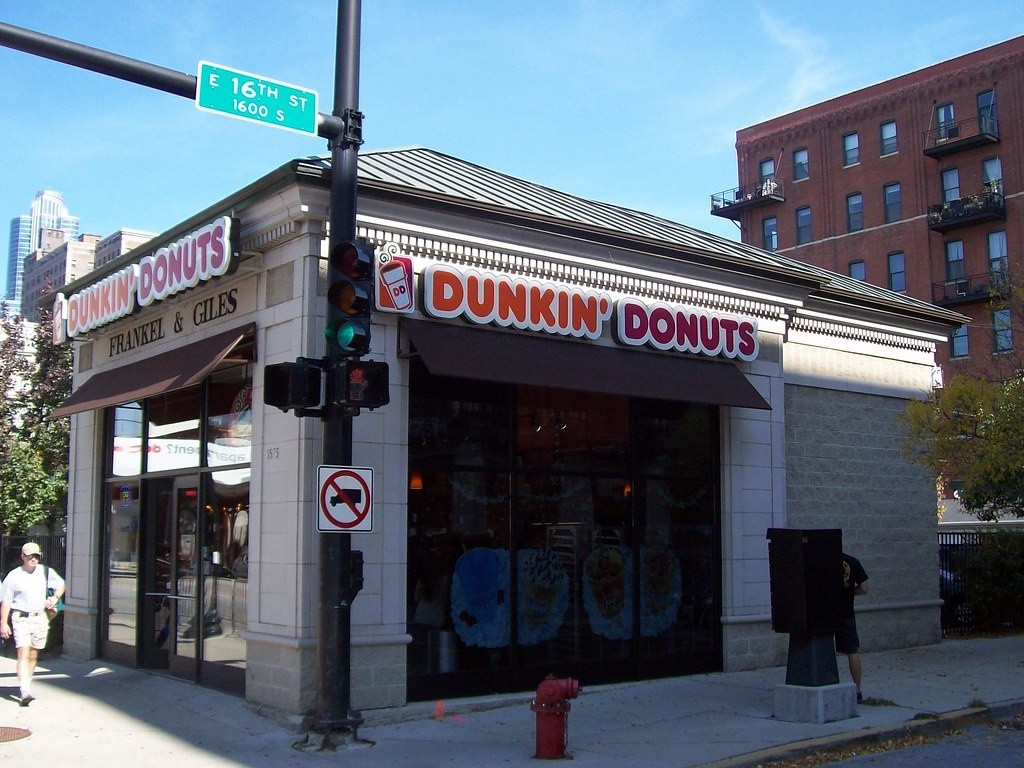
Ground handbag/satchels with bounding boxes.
[44,566,63,620]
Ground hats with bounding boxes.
[21,542,42,555]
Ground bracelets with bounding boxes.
[54,595,58,598]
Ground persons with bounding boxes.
[155,559,188,649]
[0,543,65,704]
[834,554,868,700]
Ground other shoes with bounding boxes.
[21,693,35,706]
[857,691,863,703]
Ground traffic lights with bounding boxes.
[263,361,322,413]
[346,361,390,411]
[326,236,375,357]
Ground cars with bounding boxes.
[940,570,980,626]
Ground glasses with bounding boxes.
[28,554,39,559]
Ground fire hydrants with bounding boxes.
[530,674,582,758]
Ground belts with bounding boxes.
[19,611,38,617]
